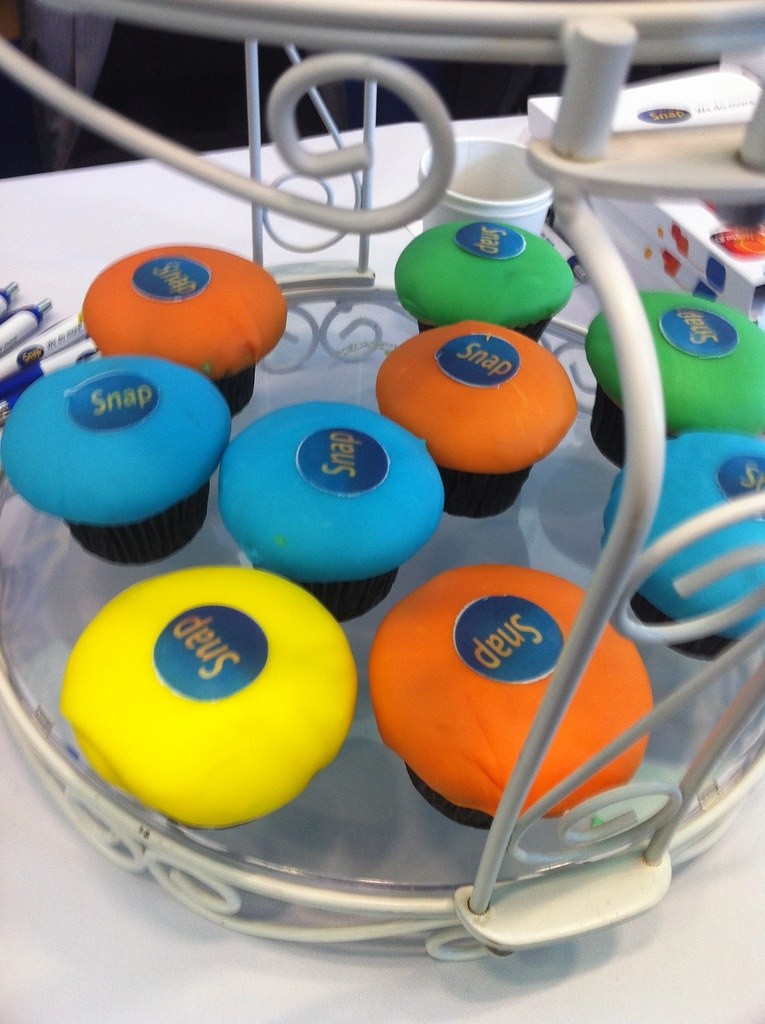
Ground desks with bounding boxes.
[1,113,765,1024]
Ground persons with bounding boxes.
[18,0,280,169]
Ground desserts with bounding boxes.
[0,221,765,834]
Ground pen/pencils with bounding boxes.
[0,281,101,426]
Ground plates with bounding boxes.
[0,291,765,901]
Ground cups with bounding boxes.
[416,138,552,238]
